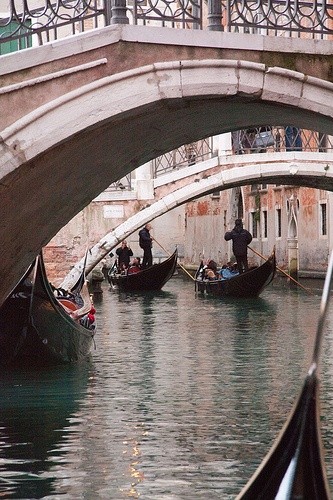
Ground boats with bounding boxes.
[106,242,178,292]
[1,249,98,363]
[193,243,278,298]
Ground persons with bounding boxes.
[139,224,155,270]
[224,218,252,274]
[196,258,223,280]
[116,240,141,274]
